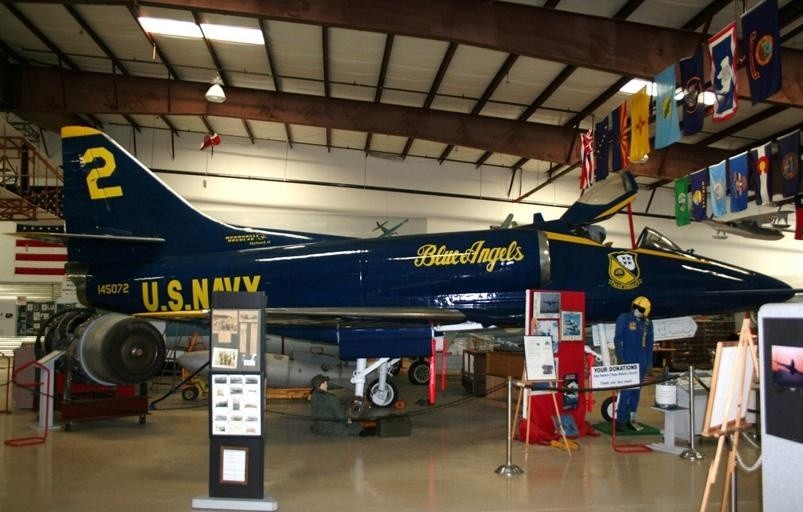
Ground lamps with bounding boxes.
[206,71,227,103]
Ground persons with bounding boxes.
[219,351,236,365]
[215,317,238,332]
[615,296,652,432]
[310,375,376,437]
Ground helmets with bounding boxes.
[631,296,652,317]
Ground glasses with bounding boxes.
[633,304,645,313]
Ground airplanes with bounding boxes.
[0,121,801,426]
[542,300,559,305]
[370,214,412,239]
[486,211,515,234]
[197,132,221,153]
[694,208,794,241]
[771,358,802,378]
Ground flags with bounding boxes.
[679,48,703,136]
[629,86,650,161]
[740,0,780,106]
[594,117,608,181]
[14,223,69,275]
[708,160,726,217]
[777,130,801,197]
[674,175,690,226]
[728,152,748,212]
[578,129,595,188]
[610,101,629,171]
[705,21,740,123]
[752,141,773,206]
[654,65,677,148]
[691,168,706,222]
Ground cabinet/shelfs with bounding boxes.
[647,404,689,457]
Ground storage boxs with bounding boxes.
[376,414,411,437]
